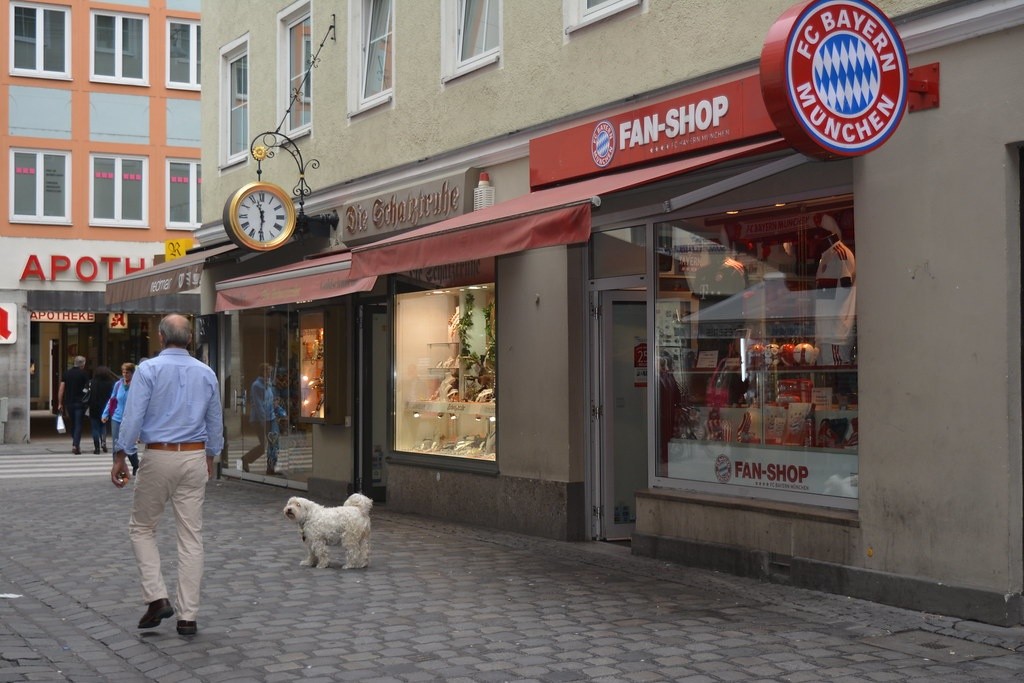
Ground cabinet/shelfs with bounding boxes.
[667,315,859,499]
[295,304,347,426]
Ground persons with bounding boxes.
[439,370,455,401]
[691,242,748,359]
[240,363,288,480]
[783,211,858,366]
[108,312,228,638]
[446,304,461,368]
[101,362,139,478]
[485,417,496,454]
[56,356,89,456]
[88,364,113,455]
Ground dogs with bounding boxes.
[283,493,373,569]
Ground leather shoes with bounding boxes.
[138,599,174,628]
[177,620,197,634]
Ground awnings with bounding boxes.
[104,243,247,308]
[211,248,377,313]
[25,290,110,313]
[348,132,790,282]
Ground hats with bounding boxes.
[820,215,841,239]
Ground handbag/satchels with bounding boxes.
[109,384,120,416]
[82,380,91,405]
[703,359,729,408]
[56,408,66,434]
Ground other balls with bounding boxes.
[747,342,813,365]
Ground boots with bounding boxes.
[100,433,107,452]
[93,437,100,454]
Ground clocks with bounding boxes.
[222,179,297,253]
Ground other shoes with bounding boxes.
[72,446,81,454]
[241,456,249,472]
[266,468,277,474]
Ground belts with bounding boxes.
[148,442,205,451]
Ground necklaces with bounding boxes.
[448,312,459,326]
[488,431,496,438]
